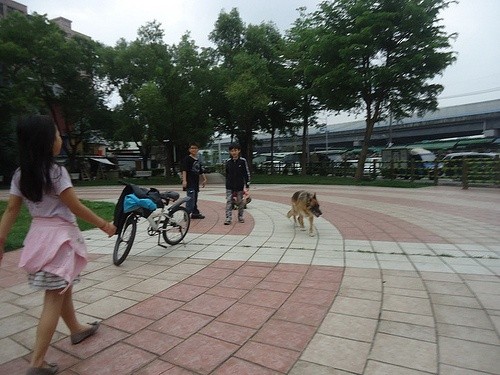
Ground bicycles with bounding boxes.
[112,180,194,266]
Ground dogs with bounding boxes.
[287,189,323,237]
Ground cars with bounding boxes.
[197,149,379,177]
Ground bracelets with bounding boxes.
[101,222,110,231]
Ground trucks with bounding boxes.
[380,146,441,182]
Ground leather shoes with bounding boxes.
[26,360,58,375]
[71,322,100,344]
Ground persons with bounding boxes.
[0,115,118,375]
[224,142,251,225]
[180,142,207,219]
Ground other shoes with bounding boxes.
[224,218,232,225]
[239,216,245,223]
[191,214,205,219]
[184,213,191,221]
